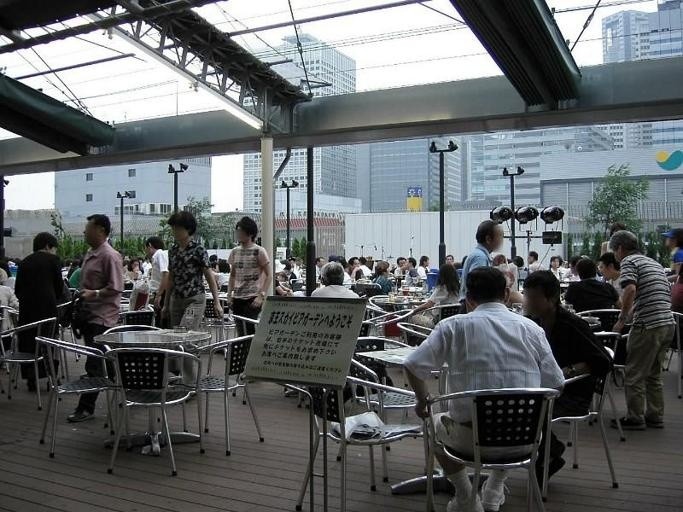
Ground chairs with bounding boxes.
[180,335,265,458]
[102,325,190,437]
[316,270,681,403]
[548,346,621,490]
[104,347,206,478]
[331,374,438,494]
[421,387,559,511]
[0,317,62,409]
[34,335,133,459]
[1,269,307,381]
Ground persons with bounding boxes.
[0,210,683,512]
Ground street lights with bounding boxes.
[354,243,378,256]
[116,191,133,252]
[278,178,300,259]
[501,164,524,258]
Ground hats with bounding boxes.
[662,228,683,238]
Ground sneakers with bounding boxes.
[69,409,94,422]
[481,480,505,512]
[446,495,483,511]
[611,415,664,430]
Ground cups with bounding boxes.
[356,273,371,284]
[388,278,422,302]
[511,302,522,314]
[561,304,572,314]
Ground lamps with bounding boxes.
[281,180,299,259]
[117,191,131,241]
[503,167,525,259]
[83,12,264,130]
